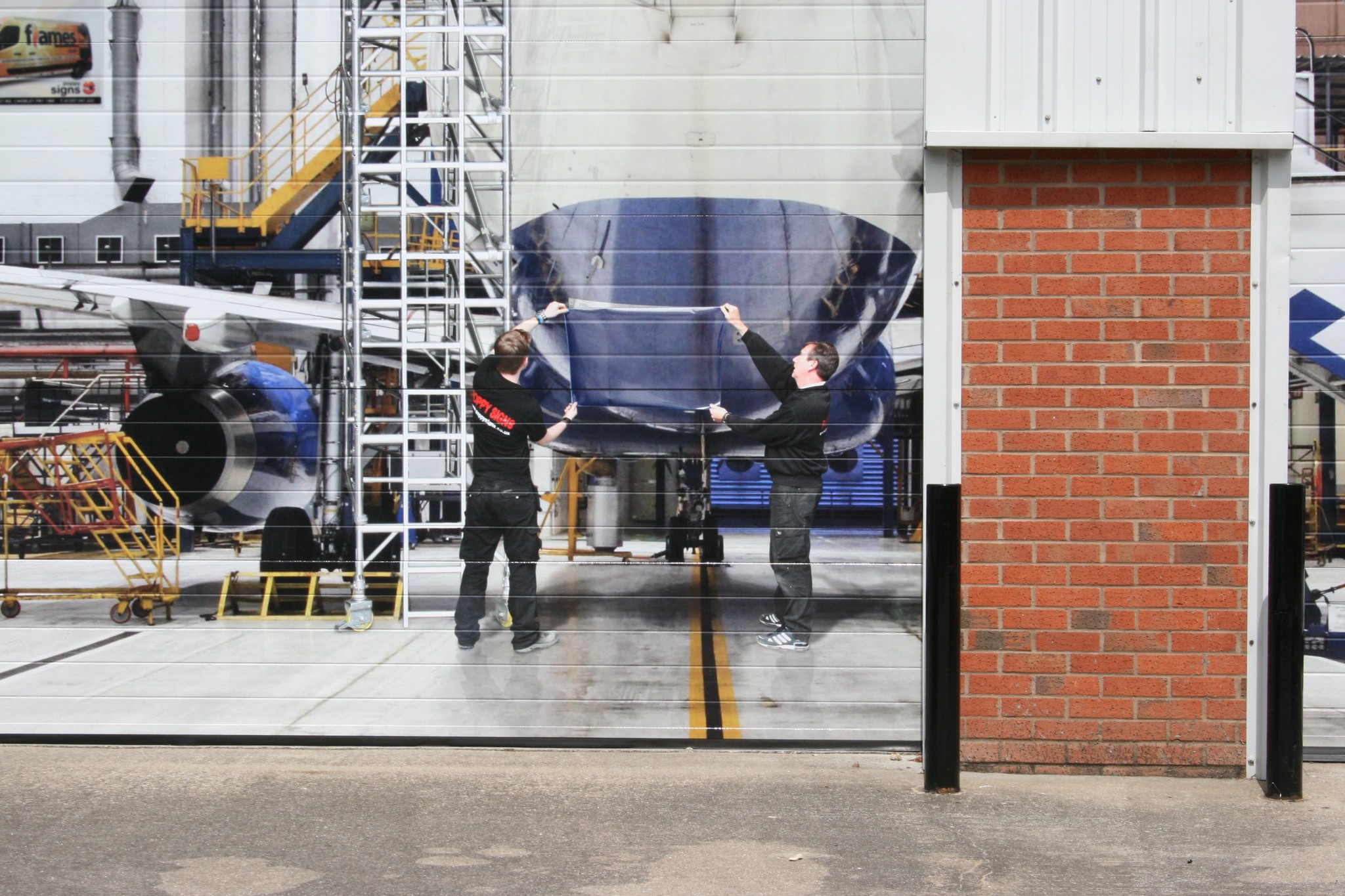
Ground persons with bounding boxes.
[450,301,595,653]
[709,302,840,652]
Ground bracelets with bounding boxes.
[535,314,544,324]
[562,417,571,426]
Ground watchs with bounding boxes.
[538,309,549,323]
[722,412,732,423]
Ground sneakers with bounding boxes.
[756,627,809,651]
[458,642,475,649]
[513,632,559,652]
[759,612,789,629]
